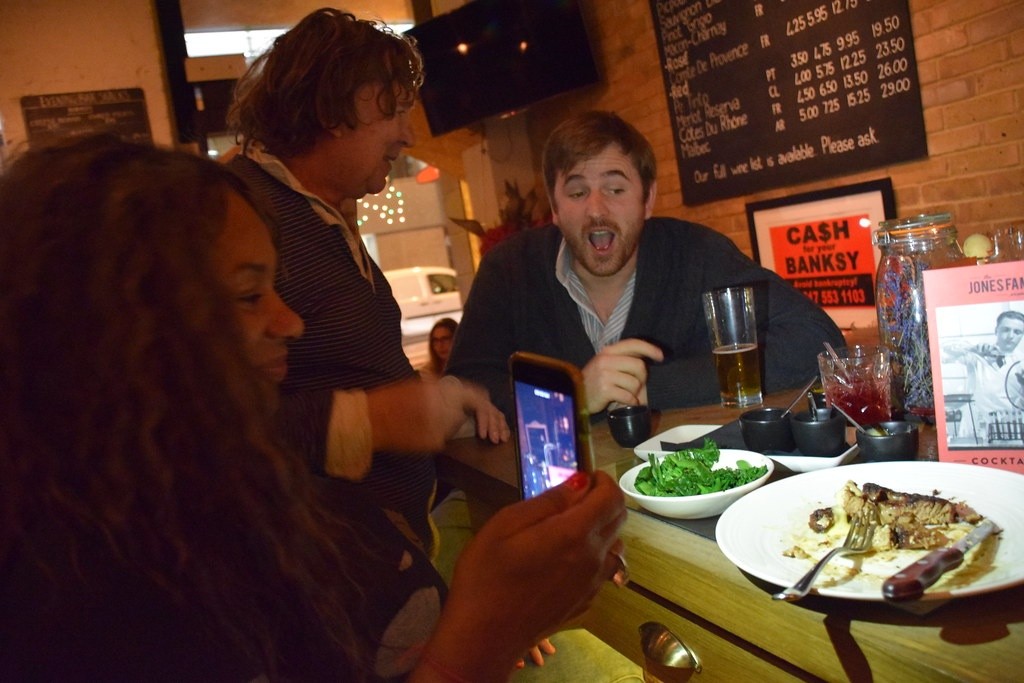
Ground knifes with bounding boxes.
[883,517,994,600]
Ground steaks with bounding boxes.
[842,479,958,526]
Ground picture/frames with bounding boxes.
[743,176,896,332]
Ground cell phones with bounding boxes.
[510,351,595,502]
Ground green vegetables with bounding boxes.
[634,438,768,497]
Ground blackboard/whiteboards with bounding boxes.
[650,1,931,208]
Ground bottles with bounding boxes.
[874,212,970,425]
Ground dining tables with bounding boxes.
[433,389,1024,682]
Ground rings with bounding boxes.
[608,548,630,591]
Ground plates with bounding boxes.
[619,449,774,519]
[634,424,857,471]
[716,460,1024,600]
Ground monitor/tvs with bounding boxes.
[401,0,601,139]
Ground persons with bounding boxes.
[942,311,1024,437]
[443,108,849,426]
[417,317,458,378]
[212,8,511,564]
[0,129,630,683]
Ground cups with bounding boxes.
[608,405,649,447]
[856,422,919,464]
[702,286,763,408]
[818,344,892,423]
[739,408,796,455]
[977,227,1024,266]
[795,408,846,457]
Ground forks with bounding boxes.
[773,513,877,600]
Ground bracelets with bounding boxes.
[420,650,467,683]
